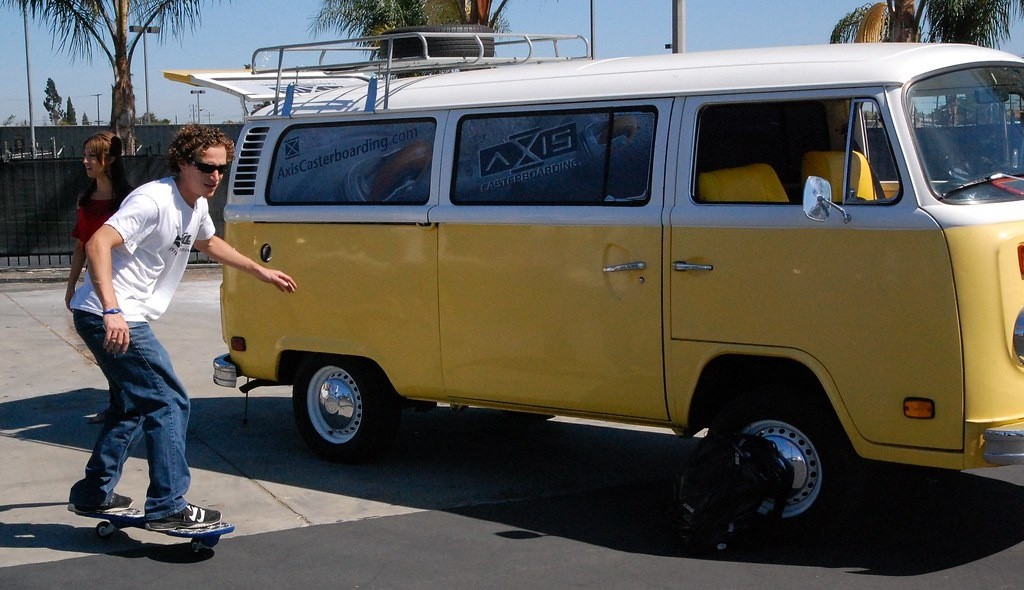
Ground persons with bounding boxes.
[65,123,297,530]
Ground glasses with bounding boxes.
[185,158,230,174]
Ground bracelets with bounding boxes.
[102,308,123,314]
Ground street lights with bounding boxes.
[90,94,103,126]
[128,25,161,123]
[190,89,209,124]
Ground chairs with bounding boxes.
[697,163,790,202]
[801,151,874,202]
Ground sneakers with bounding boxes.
[68,493,132,514]
[145,503,221,531]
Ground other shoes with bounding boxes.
[88,409,109,424]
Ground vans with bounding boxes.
[166,28,1024,530]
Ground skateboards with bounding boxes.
[67,503,235,554]
